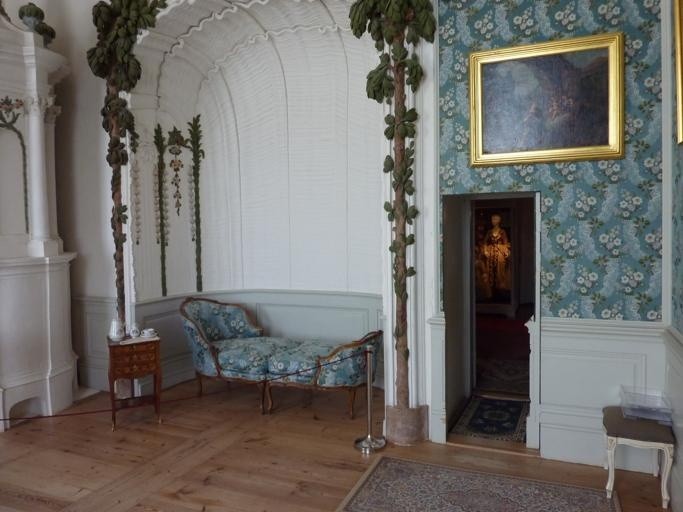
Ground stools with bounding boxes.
[601,406,675,508]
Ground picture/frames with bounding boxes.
[472,205,519,318]
[469,31,625,167]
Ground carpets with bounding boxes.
[335,456,622,512]
[448,395,530,447]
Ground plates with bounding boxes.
[141,334,158,338]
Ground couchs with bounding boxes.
[181,298,300,414]
[266,330,384,419]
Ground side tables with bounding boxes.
[106,334,161,432]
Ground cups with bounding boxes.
[142,328,159,336]
